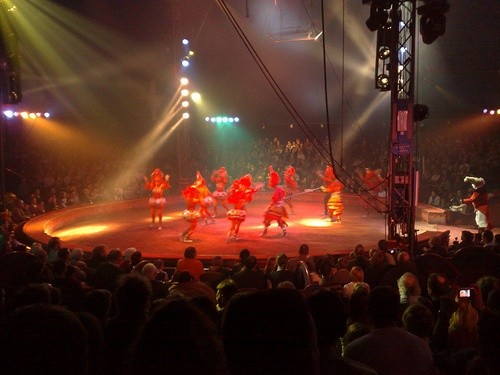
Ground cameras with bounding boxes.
[458,288,474,300]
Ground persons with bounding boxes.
[144,168,170,229]
[0,135,500,375]
